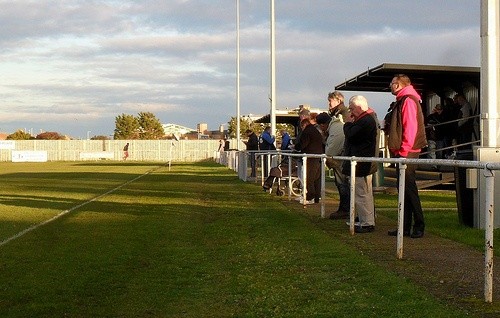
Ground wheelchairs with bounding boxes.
[269,163,304,197]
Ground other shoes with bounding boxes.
[388,229,410,236]
[300,198,315,204]
[346,220,360,226]
[330,210,350,219]
[356,226,374,232]
[410,229,424,238]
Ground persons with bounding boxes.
[224,139,230,151]
[123,142,129,160]
[258,126,276,177]
[387,73,427,237]
[243,129,259,177]
[342,95,380,233]
[293,109,324,202]
[280,129,291,162]
[289,118,324,205]
[323,91,354,123]
[383,91,473,169]
[218,140,224,151]
[316,112,352,219]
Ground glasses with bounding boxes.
[390,83,397,85]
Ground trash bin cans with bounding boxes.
[373,150,384,187]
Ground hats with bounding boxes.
[297,109,311,116]
